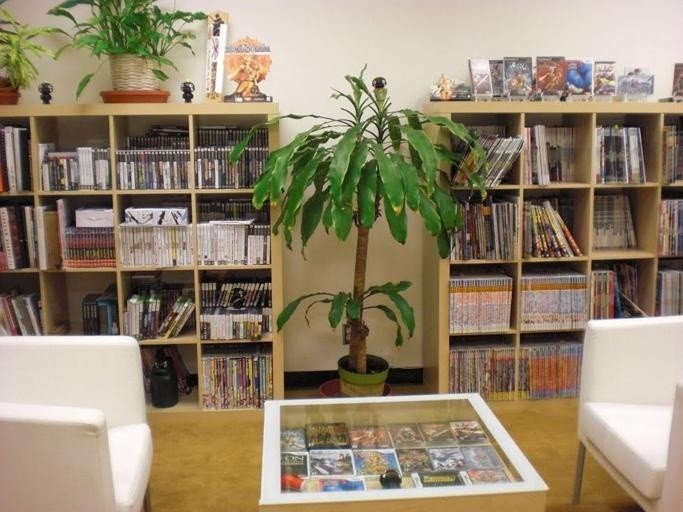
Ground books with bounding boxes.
[449,54,682,399]
[279,419,510,493]
[0,127,273,407]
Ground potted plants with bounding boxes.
[229,62,488,399]
[0,2,216,105]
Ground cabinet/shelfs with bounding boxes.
[421,98,682,407]
[1,103,286,412]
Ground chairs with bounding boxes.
[0,336,151,511]
[570,314,683,512]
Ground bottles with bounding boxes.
[149,358,178,409]
